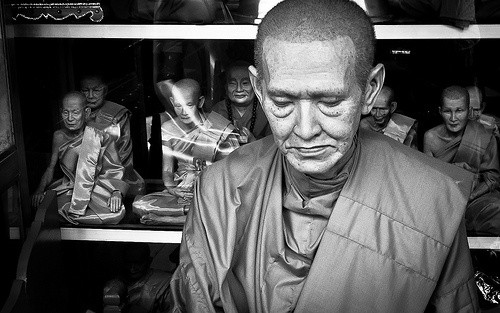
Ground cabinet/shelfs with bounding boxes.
[0,0,500,313]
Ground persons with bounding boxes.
[30,73,500,231]
[171,1,481,313]
